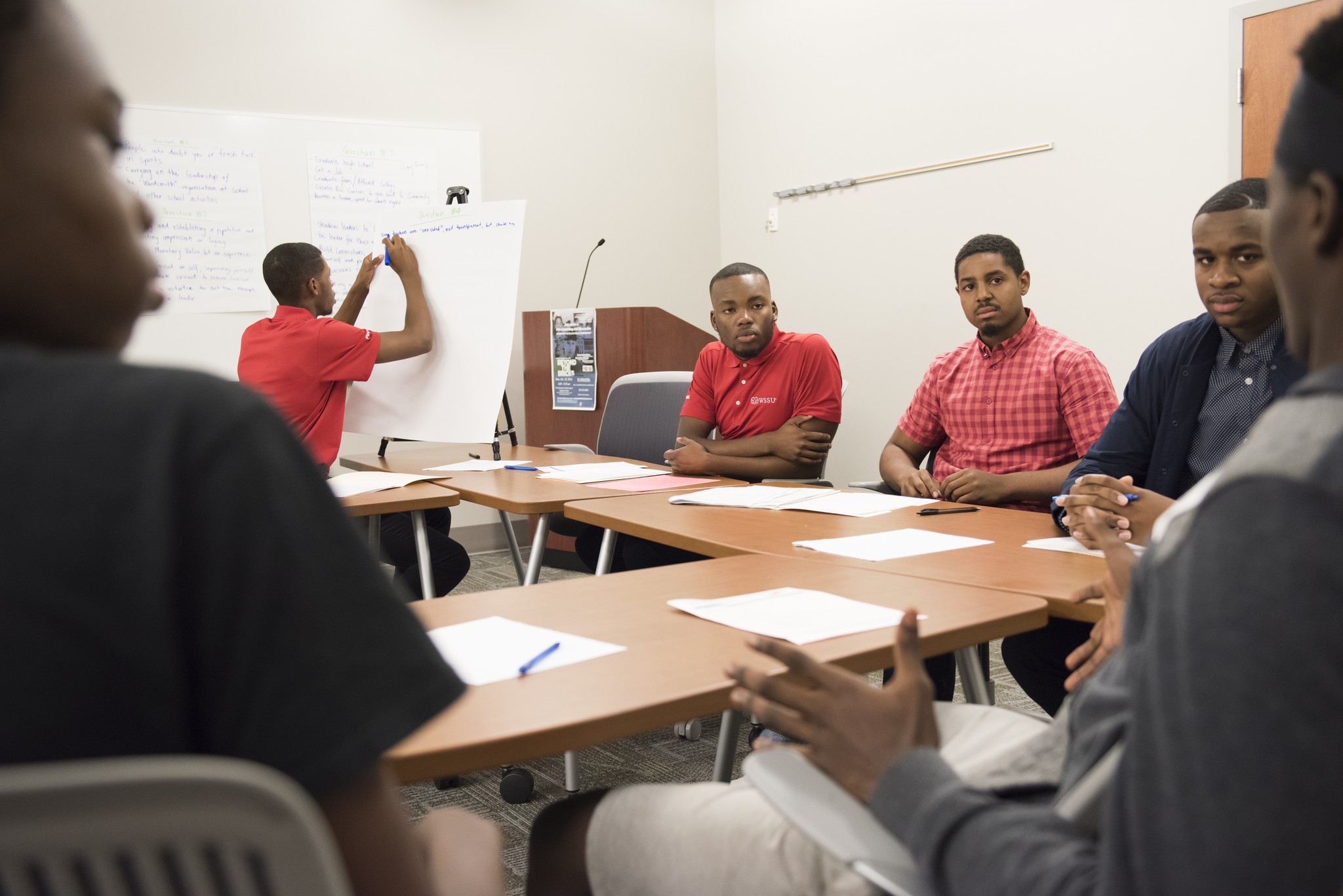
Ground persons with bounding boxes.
[527,7,1343,896]
[666,177,1301,721]
[0,1,505,896]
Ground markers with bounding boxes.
[469,453,480,458]
[385,234,390,266]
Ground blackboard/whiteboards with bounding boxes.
[40,103,480,382]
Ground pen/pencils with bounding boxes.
[504,465,538,472]
[519,643,560,672]
[1052,494,1137,500]
[917,507,981,515]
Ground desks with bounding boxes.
[325,440,1103,794]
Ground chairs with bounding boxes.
[0,756,347,896]
[522,371,722,584]
